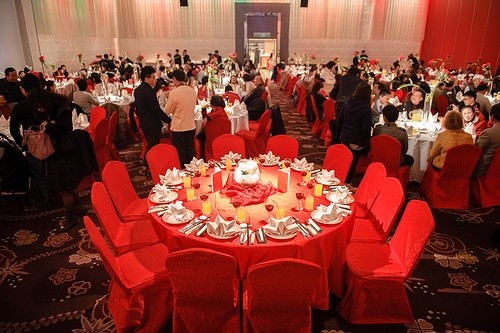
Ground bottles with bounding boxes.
[118,84,121,96]
[225,94,229,106]
[402,103,407,121]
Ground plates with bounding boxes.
[149,166,355,239]
[222,159,238,166]
[262,162,279,166]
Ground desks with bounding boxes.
[395,121,437,183]
[194,105,249,135]
[147,158,354,310]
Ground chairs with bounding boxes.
[0,69,500,333]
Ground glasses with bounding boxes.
[146,76,156,79]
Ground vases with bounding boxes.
[423,95,433,120]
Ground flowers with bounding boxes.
[39,55,48,74]
[291,52,500,104]
[110,46,237,69]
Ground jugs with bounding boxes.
[409,109,421,122]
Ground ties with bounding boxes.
[380,105,383,114]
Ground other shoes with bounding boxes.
[59,215,79,233]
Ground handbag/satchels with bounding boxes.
[22,130,55,160]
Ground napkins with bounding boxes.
[206,215,241,237]
[152,182,168,198]
[317,167,337,184]
[335,185,353,202]
[184,155,204,171]
[167,200,188,220]
[258,150,280,164]
[290,157,314,170]
[312,202,344,222]
[159,166,179,183]
[220,151,241,164]
[75,113,88,124]
[262,215,299,236]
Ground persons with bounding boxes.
[0,50,500,201]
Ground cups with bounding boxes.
[305,195,314,211]
[184,177,190,188]
[186,189,194,201]
[281,161,290,169]
[303,169,311,181]
[315,182,322,197]
[237,209,247,223]
[200,165,206,176]
[276,207,285,220]
[226,159,232,170]
[202,201,211,215]
